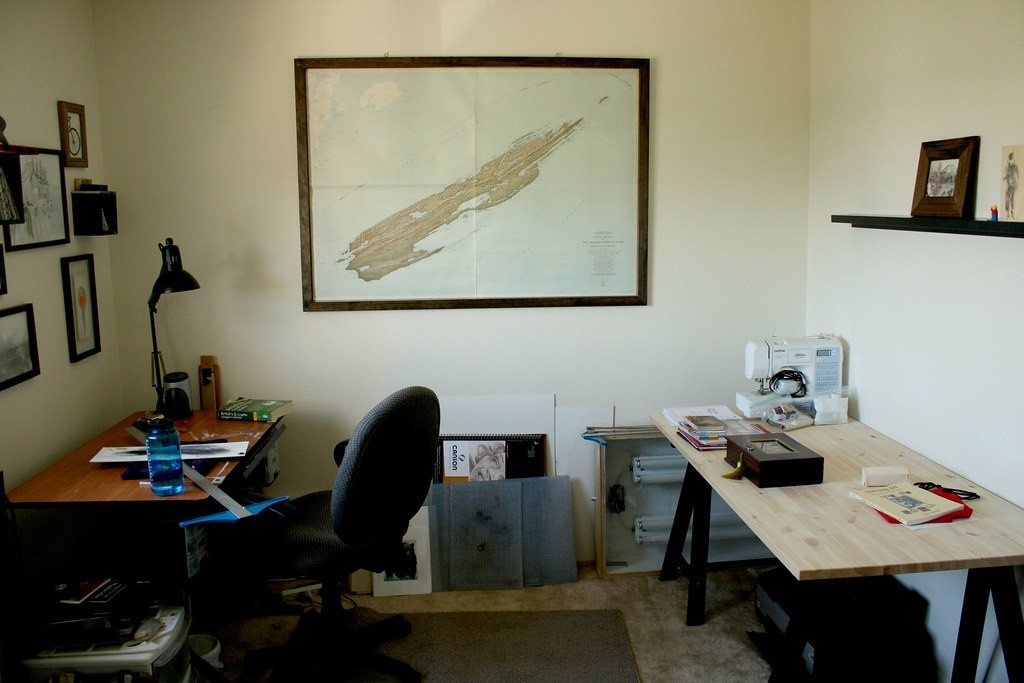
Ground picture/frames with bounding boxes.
[0,304,41,390]
[61,252,102,363]
[292,54,651,306]
[909,134,981,218]
[56,97,89,169]
[1,146,69,251]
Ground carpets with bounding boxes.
[287,606,640,683]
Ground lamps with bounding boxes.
[144,237,201,420]
[630,457,689,485]
[633,515,757,547]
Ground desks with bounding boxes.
[3,410,284,683]
[650,408,1024,683]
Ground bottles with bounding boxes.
[145,417,186,497]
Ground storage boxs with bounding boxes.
[722,433,824,489]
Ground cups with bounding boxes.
[163,372,193,419]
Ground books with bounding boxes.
[849,481,965,525]
[216,397,294,421]
[676,415,771,452]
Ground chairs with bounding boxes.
[230,385,441,683]
